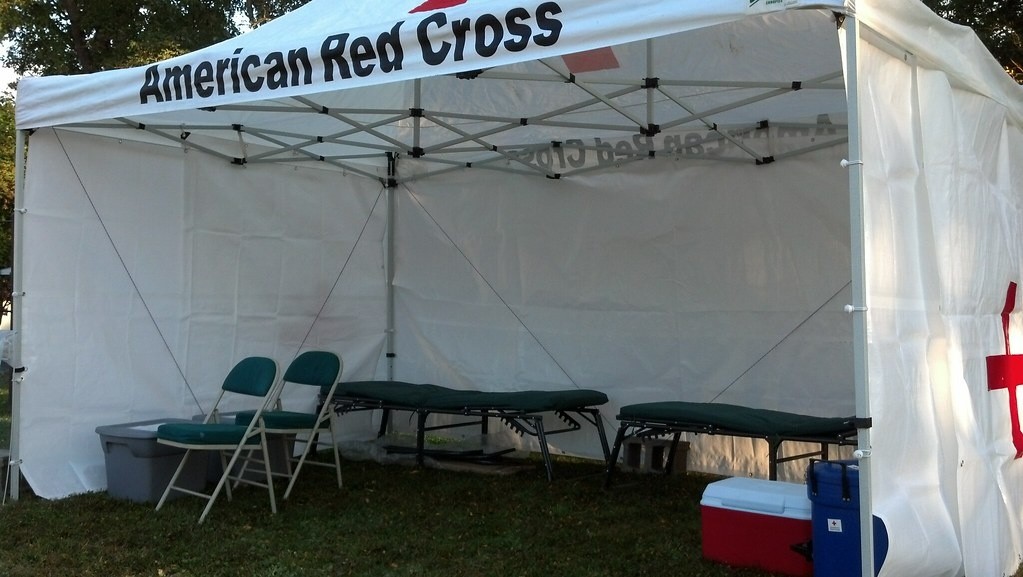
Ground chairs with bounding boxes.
[153,356,278,523]
[231,349,344,501]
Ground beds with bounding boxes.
[312,380,610,483]
[609,402,857,480]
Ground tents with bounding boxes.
[9,0,1023,577]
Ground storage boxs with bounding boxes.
[699,477,812,575]
[191,409,298,486]
[95,417,212,510]
